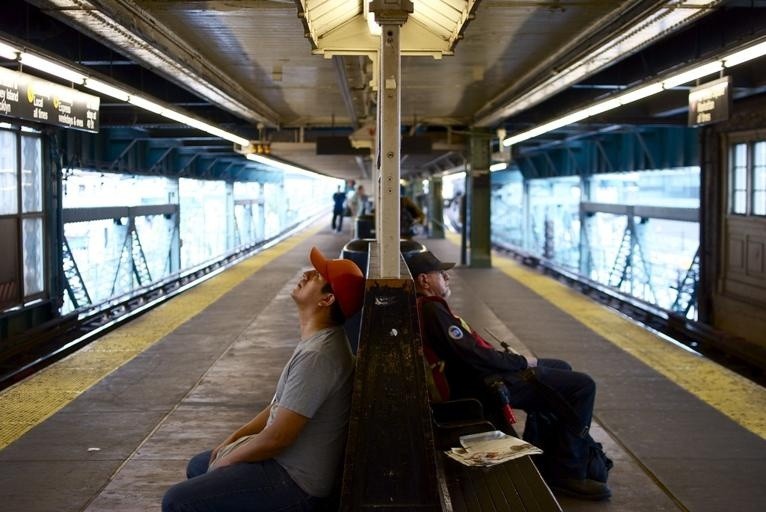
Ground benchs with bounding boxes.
[398,280,560,512]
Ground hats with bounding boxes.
[307,245,364,320]
[400,240,455,275]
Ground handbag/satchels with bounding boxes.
[342,207,352,216]
[400,224,423,236]
[588,439,612,484]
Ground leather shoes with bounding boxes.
[542,472,611,500]
